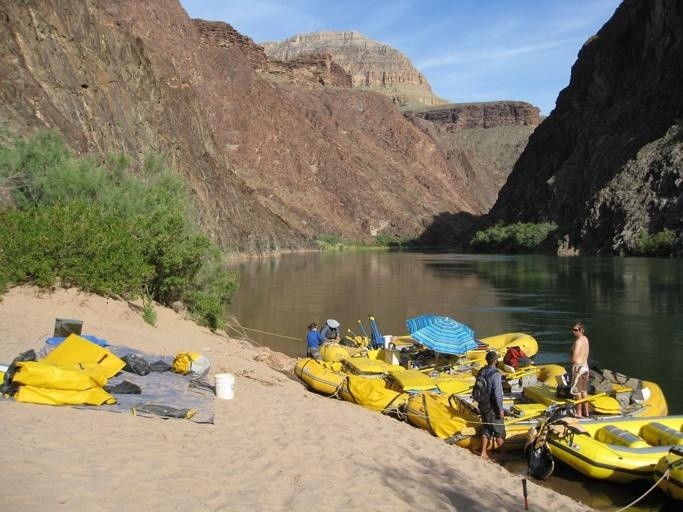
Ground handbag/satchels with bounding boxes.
[503,348,531,369]
[172,352,211,378]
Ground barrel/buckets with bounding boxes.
[214,374,235,400]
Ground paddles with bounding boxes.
[461,385,632,436]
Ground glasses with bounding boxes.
[572,328,580,333]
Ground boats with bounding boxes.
[293,332,683,505]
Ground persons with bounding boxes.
[571,322,591,418]
[478,351,509,461]
[321,318,341,343]
[306,324,330,363]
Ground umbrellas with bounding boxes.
[367,313,385,347]
[407,312,478,356]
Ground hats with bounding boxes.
[326,319,340,329]
[486,351,501,362]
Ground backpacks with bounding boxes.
[473,367,499,404]
